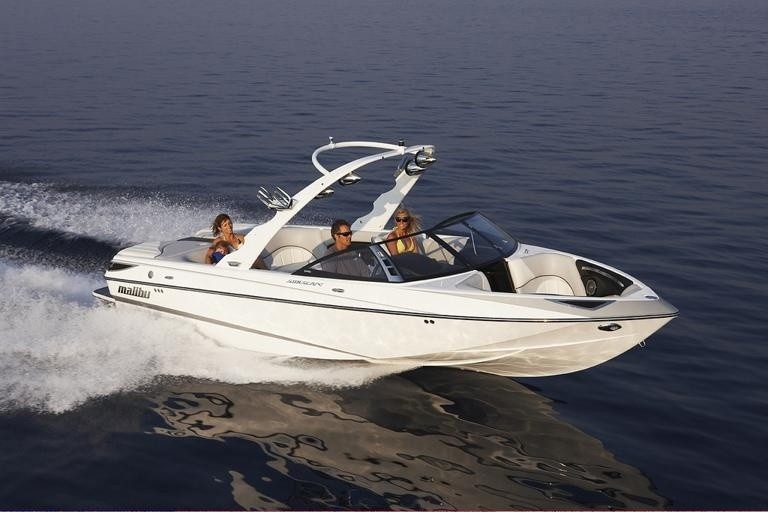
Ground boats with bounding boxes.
[132,357,670,511]
[93,134,681,380]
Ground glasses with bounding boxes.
[337,231,352,236]
[395,217,409,222]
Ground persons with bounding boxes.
[386,207,424,256]
[321,219,371,279]
[205,214,267,270]
[211,241,229,264]
